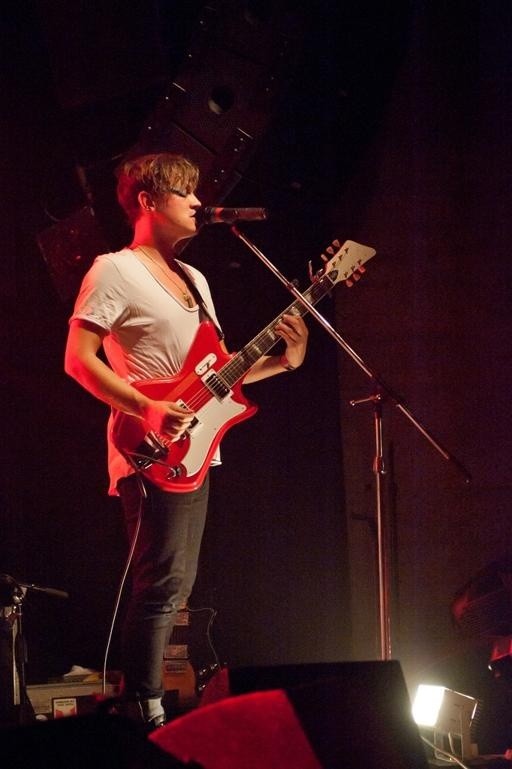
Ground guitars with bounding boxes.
[111,237,377,492]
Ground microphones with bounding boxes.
[195,204,268,225]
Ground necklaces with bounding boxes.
[138,246,194,308]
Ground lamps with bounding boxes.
[410,684,476,760]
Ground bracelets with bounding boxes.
[280,349,296,372]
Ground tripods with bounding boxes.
[224,227,476,769]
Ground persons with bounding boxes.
[58,150,310,733]
